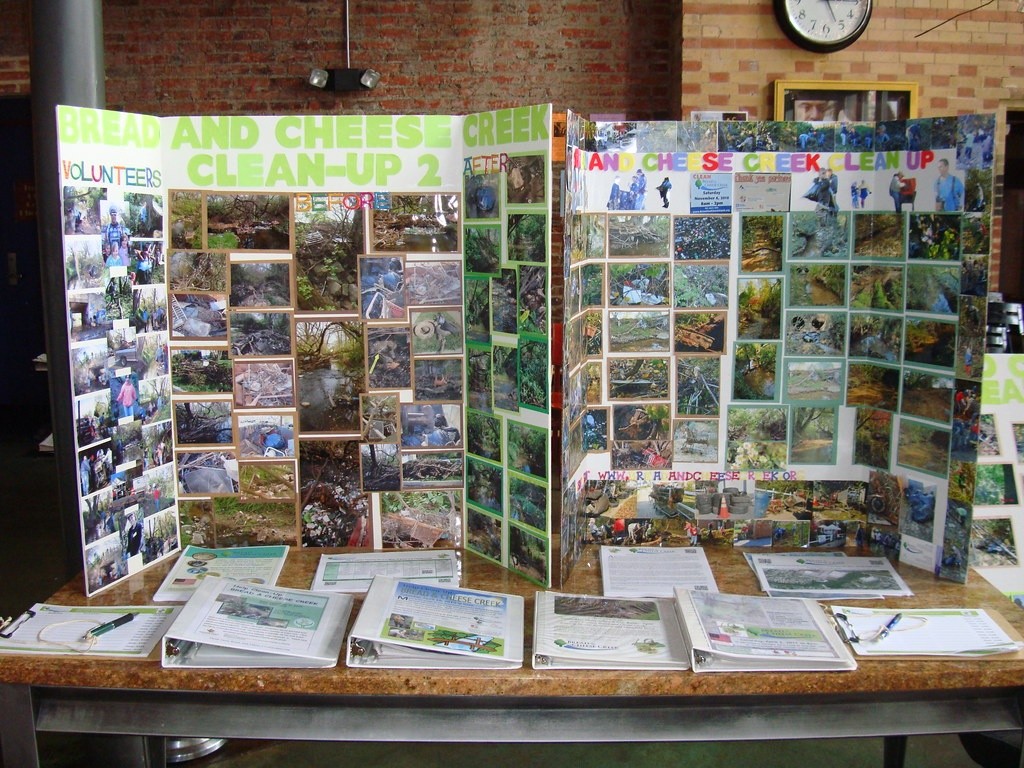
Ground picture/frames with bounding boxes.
[774,79,919,121]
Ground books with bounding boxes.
[0,544,1024,669]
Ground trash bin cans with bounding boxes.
[755,492,769,517]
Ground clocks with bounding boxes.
[772,0,873,54]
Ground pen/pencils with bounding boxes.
[83,611,140,641]
[876,612,904,642]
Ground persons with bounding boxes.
[54,100,1020,583]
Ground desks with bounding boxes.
[0,534,1024,768]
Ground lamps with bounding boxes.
[309,0,382,92]
